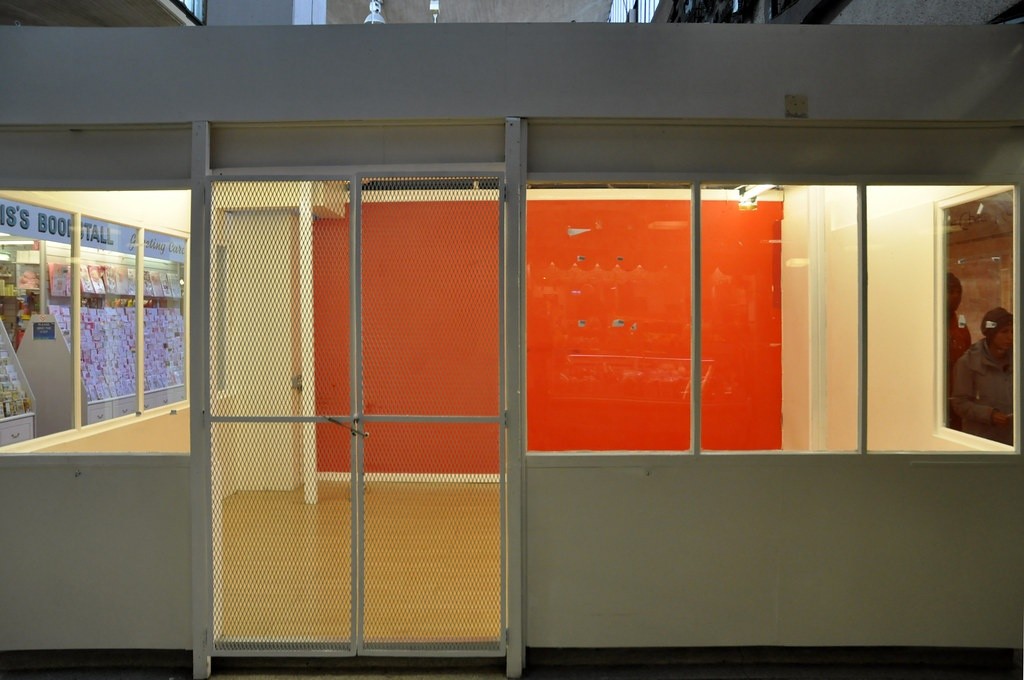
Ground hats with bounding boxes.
[980,308,1014,335]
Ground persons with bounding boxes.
[951,306,1013,447]
[946,272,972,432]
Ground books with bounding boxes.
[48,304,185,402]
[48,262,182,300]
[0,327,32,418]
